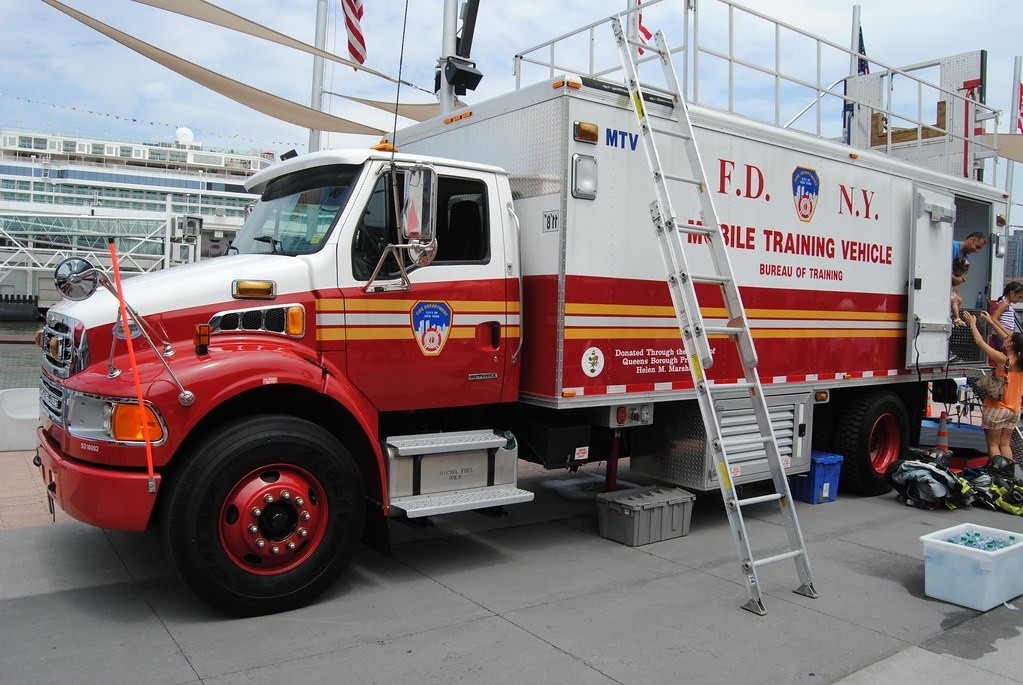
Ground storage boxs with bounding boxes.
[595,485,696,546]
[919,522,1023,612]
[770,450,844,505]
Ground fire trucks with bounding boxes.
[32,0,1023,622]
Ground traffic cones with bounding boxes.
[925,383,932,416]
[936,411,949,453]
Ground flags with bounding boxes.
[1017,82,1023,133]
[341,0,366,72]
[638,0,652,56]
[858,26,869,74]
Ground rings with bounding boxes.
[965,317,967,319]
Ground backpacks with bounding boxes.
[890,453,977,510]
[960,466,1023,515]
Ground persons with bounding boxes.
[950,231,1023,461]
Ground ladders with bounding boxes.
[612,13,822,616]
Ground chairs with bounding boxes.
[442,201,482,260]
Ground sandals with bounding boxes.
[954,320,967,326]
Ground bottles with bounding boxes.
[946,529,1016,552]
[976,291,983,311]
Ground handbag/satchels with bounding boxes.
[976,356,1009,400]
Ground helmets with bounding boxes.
[985,455,1014,476]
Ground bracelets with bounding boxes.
[969,326,976,329]
